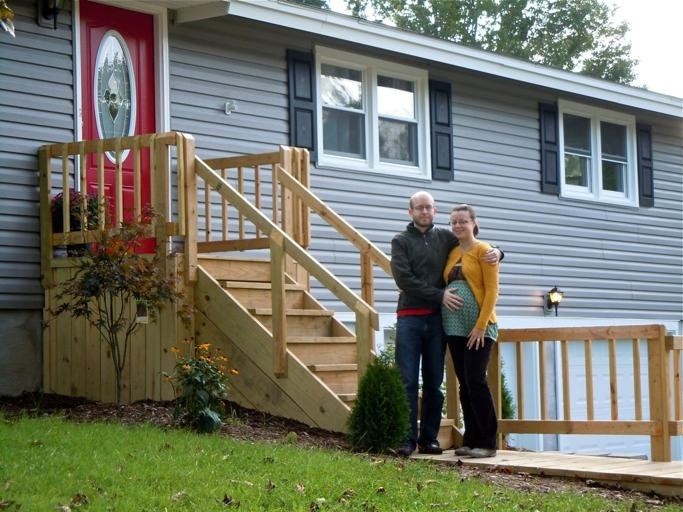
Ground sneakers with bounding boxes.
[418,442,441,454]
[398,442,415,455]
[455,445,471,455]
[470,448,496,457]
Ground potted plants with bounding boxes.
[48,186,114,259]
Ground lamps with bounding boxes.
[36,0,67,31]
[545,284,565,315]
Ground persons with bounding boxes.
[388,189,504,457]
[439,203,501,458]
